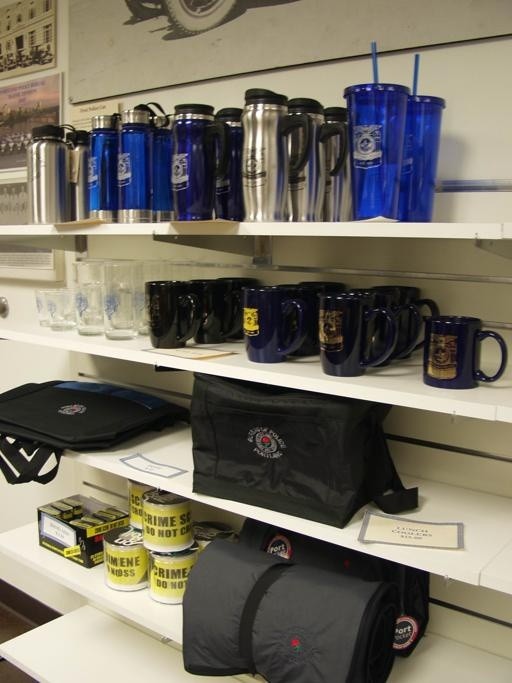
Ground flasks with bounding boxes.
[28,85,350,221]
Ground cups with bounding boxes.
[423,313,508,389]
[33,255,173,344]
[345,85,444,222]
[148,277,436,375]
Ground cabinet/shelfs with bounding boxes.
[0,216,512,682]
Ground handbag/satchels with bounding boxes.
[191,373,419,530]
[1,380,192,486]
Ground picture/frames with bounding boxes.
[0,70,66,284]
